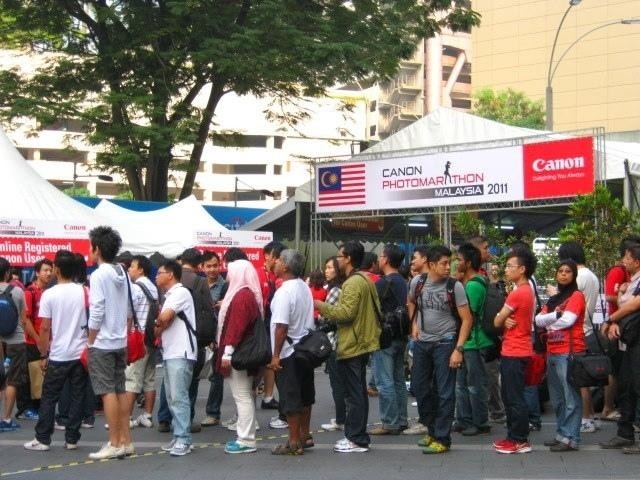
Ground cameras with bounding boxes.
[319,318,337,333]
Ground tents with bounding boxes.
[240,107,640,249]
[0,124,229,265]
[88,192,276,248]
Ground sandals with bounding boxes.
[271,444,303,455]
[286,434,314,447]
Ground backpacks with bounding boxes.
[469,275,506,336]
[0,285,19,336]
[530,277,548,354]
[177,284,214,348]
[135,280,164,364]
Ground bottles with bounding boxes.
[3,357,10,374]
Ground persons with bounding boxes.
[157,248,213,433]
[548,240,600,433]
[73,253,90,427]
[601,236,631,420]
[201,252,228,426]
[20,258,53,420]
[535,259,588,452]
[450,238,491,282]
[213,258,265,453]
[455,346,464,352]
[268,243,291,429]
[601,234,640,448]
[260,242,279,410]
[104,255,160,430]
[308,270,326,320]
[412,245,430,275]
[270,247,314,455]
[409,245,472,454]
[24,249,90,451]
[507,241,536,431]
[315,244,382,453]
[318,256,347,432]
[1,257,26,433]
[493,249,534,454]
[365,243,408,435]
[154,259,198,456]
[221,246,260,432]
[82,225,135,459]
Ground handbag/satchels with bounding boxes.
[80,284,89,369]
[223,304,272,370]
[618,310,640,346]
[528,276,547,353]
[120,264,145,362]
[585,278,606,354]
[567,293,613,386]
[273,322,330,375]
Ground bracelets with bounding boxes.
[224,352,232,357]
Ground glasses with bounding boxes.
[559,270,570,272]
[505,264,520,268]
[336,255,345,257]
[157,271,168,275]
[380,255,385,257]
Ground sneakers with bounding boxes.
[489,415,506,422]
[418,435,434,445]
[269,419,289,428]
[53,420,66,430]
[580,417,595,433]
[105,415,134,430]
[493,438,515,448]
[452,422,468,430]
[335,442,370,452]
[594,414,601,428]
[227,418,259,431]
[16,407,39,420]
[410,422,423,428]
[226,439,237,444]
[23,437,50,450]
[367,385,379,396]
[496,442,532,454]
[423,442,451,453]
[162,439,194,450]
[80,417,95,428]
[138,413,154,427]
[330,418,337,424]
[261,397,279,408]
[89,441,126,460]
[0,419,21,432]
[224,444,257,453]
[170,441,191,455]
[191,420,200,431]
[64,442,77,449]
[271,416,279,420]
[157,421,170,432]
[126,442,134,455]
[221,414,238,427]
[528,423,539,430]
[321,424,345,431]
[404,426,428,435]
[337,436,349,444]
[462,424,489,435]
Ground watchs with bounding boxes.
[604,316,611,324]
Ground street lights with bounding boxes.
[546,0,640,132]
[234,177,275,207]
[72,162,113,196]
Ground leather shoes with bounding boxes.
[623,445,640,454]
[366,426,400,435]
[201,417,220,425]
[544,438,561,445]
[599,436,634,449]
[550,442,578,452]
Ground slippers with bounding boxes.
[602,410,621,421]
